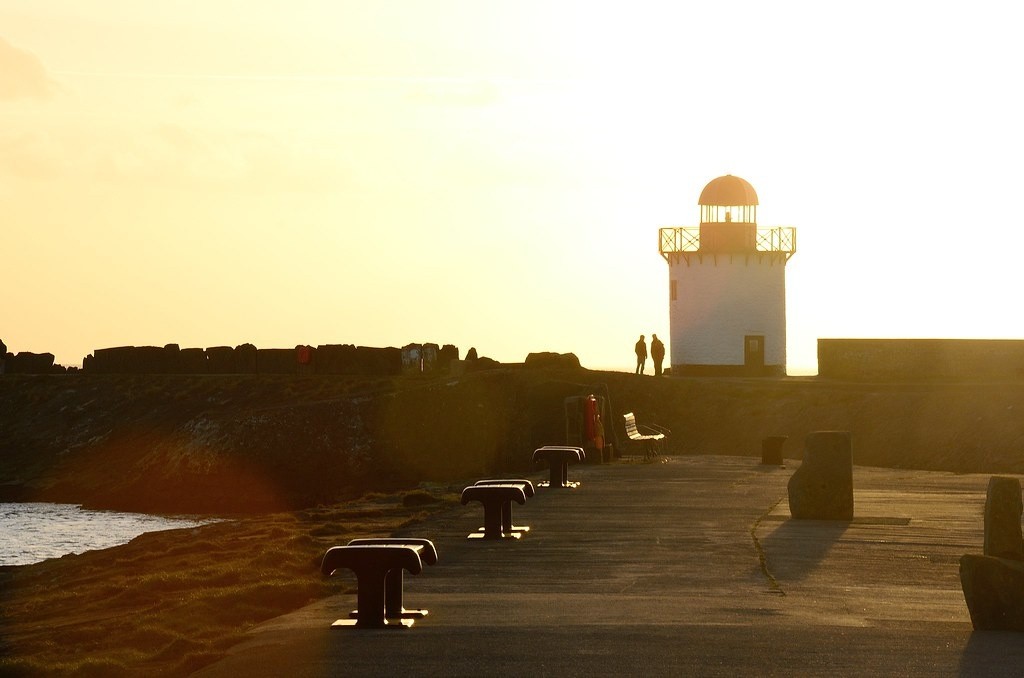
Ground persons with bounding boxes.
[635,334,648,374]
[652,333,665,376]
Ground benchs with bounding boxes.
[621,411,664,461]
[323,536,440,631]
[533,446,586,489]
[458,478,537,543]
